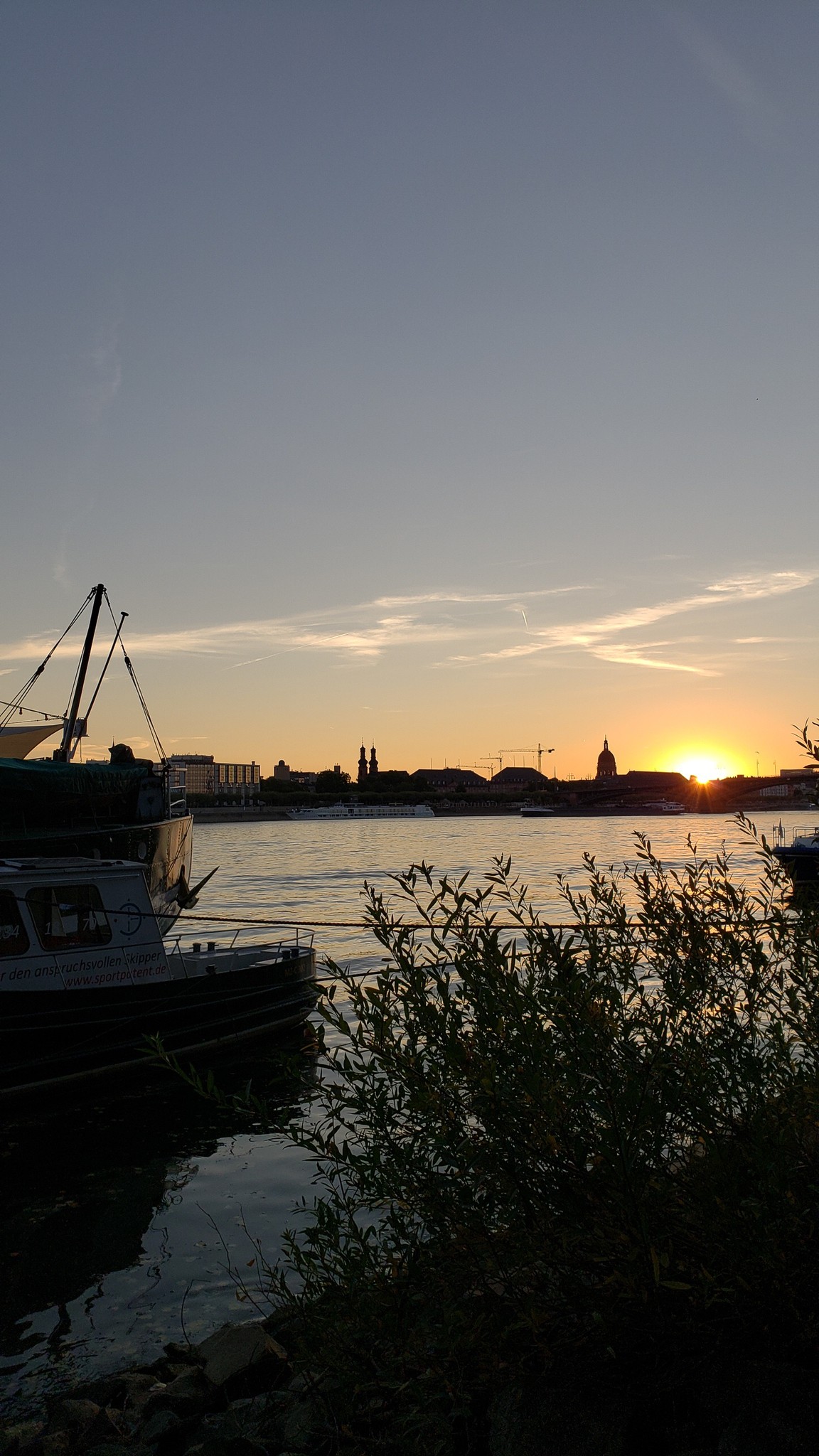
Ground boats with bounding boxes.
[0,853,326,1144]
[0,580,219,939]
[642,798,686,816]
[520,798,555,817]
[771,825,819,891]
[284,798,436,821]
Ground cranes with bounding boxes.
[498,742,555,774]
[456,762,496,781]
[479,753,504,771]
